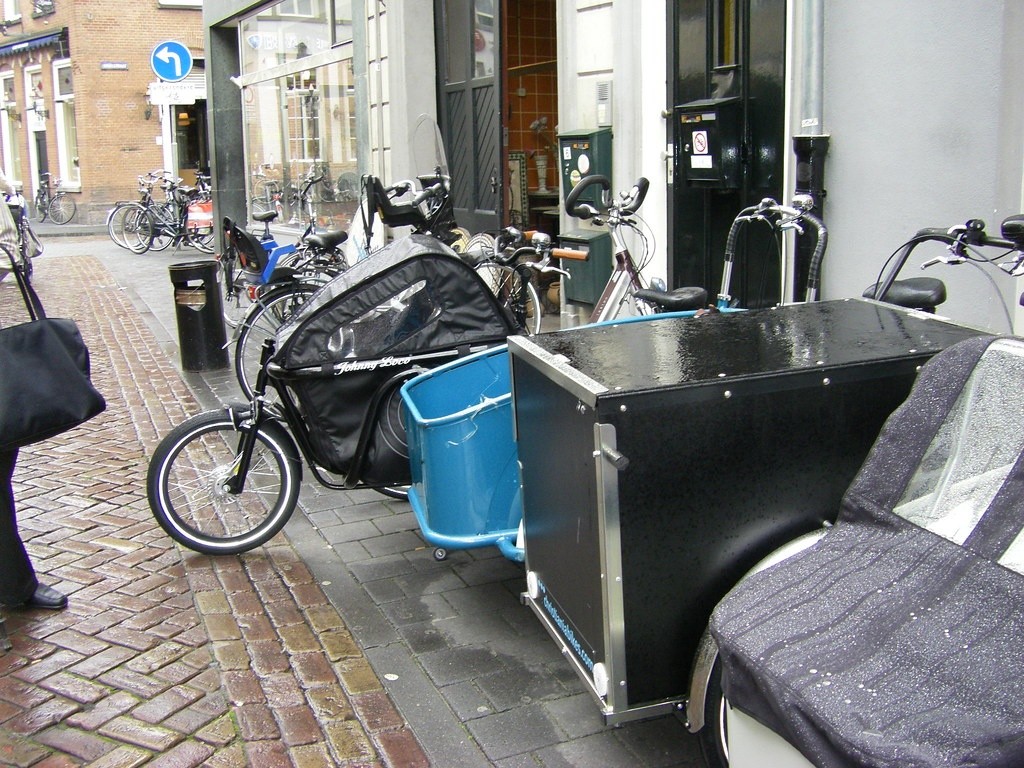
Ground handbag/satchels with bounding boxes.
[1,246,106,449]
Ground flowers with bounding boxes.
[529,116,559,152]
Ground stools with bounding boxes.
[530,205,560,288]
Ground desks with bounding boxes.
[528,188,559,200]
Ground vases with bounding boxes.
[554,152,559,174]
[535,155,550,194]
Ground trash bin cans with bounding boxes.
[168,260,230,373]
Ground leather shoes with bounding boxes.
[29,582,69,609]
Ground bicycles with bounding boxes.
[107,167,214,255]
[249,152,357,234]
[34,178,76,224]
[145,155,1024,557]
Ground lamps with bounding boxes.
[178,112,190,126]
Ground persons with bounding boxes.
[0,165,69,610]
